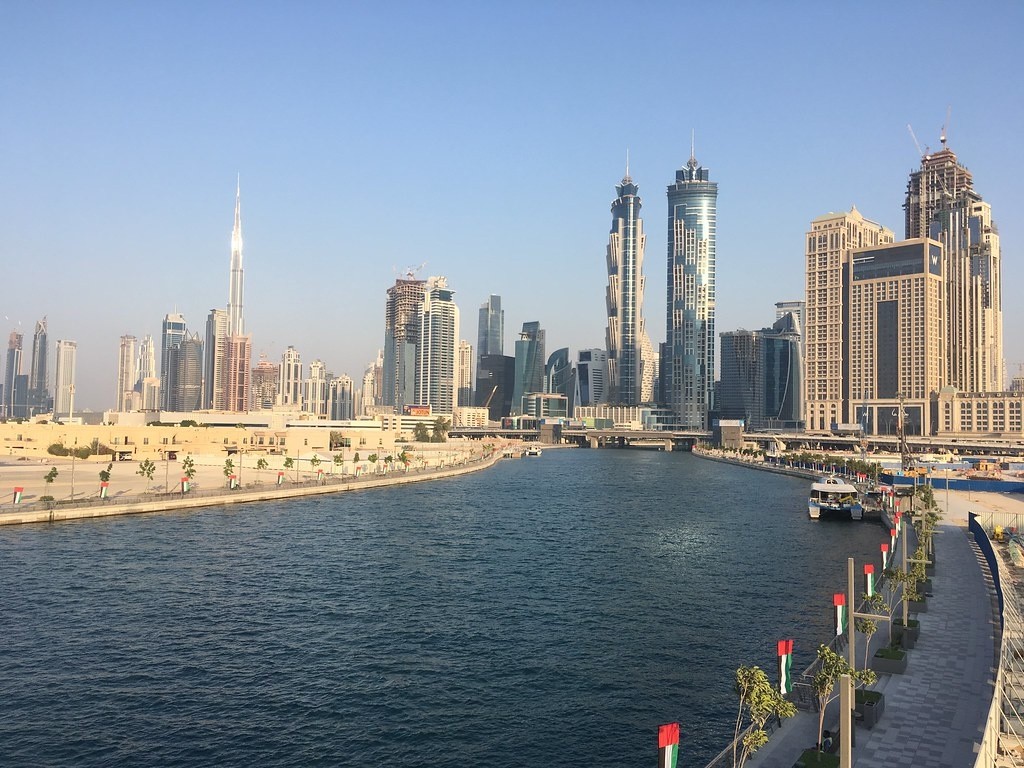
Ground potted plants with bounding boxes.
[902,563,928,613]
[915,542,935,577]
[791,644,879,768]
[892,565,923,643]
[912,549,932,592]
[855,590,892,730]
[871,562,919,673]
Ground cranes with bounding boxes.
[906,122,953,209]
[939,104,952,150]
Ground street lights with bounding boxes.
[67,383,76,424]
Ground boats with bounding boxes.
[808,475,863,521]
[525,443,543,457]
[507,445,526,458]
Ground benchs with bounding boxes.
[817,728,839,754]
